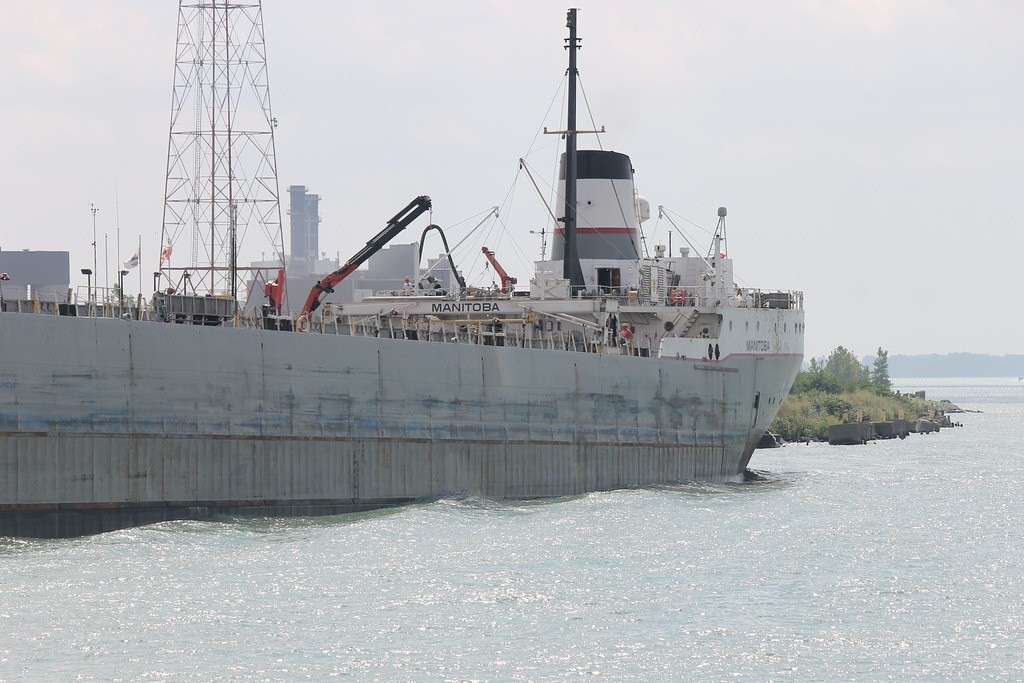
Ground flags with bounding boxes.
[124,249,138,269]
[161,244,172,259]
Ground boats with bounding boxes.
[1,2,805,505]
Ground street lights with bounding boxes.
[81,268,93,300]
[90,203,99,302]
[121,270,129,305]
[153,272,161,290]
[184,273,191,295]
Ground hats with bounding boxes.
[622,323,628,326]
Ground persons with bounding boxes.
[403,276,412,290]
[708,343,720,360]
[0,273,10,312]
[620,323,633,346]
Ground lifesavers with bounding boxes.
[296,314,308,333]
[671,288,686,303]
